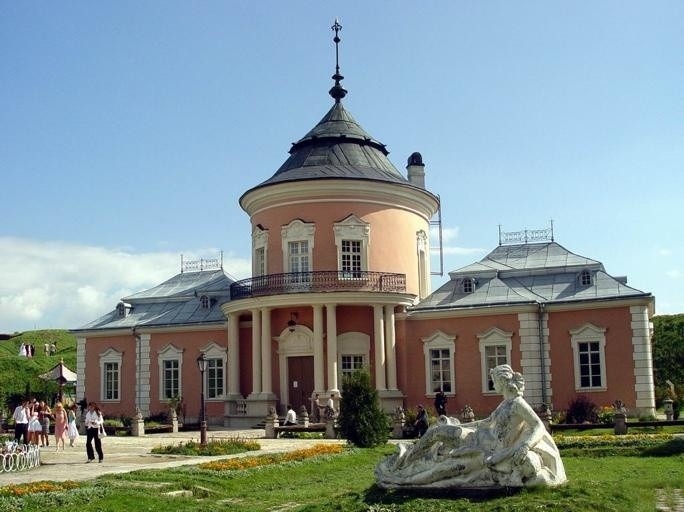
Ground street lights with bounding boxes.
[196,350,211,450]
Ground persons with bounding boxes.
[85,402,104,464]
[13,397,78,453]
[312,393,336,423]
[388,363,545,486]
[78,398,87,413]
[44,341,57,356]
[18,342,35,357]
[279,404,296,425]
[435,392,447,416]
[413,405,429,438]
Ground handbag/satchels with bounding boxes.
[98,423,107,439]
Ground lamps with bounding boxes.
[287,311,299,333]
